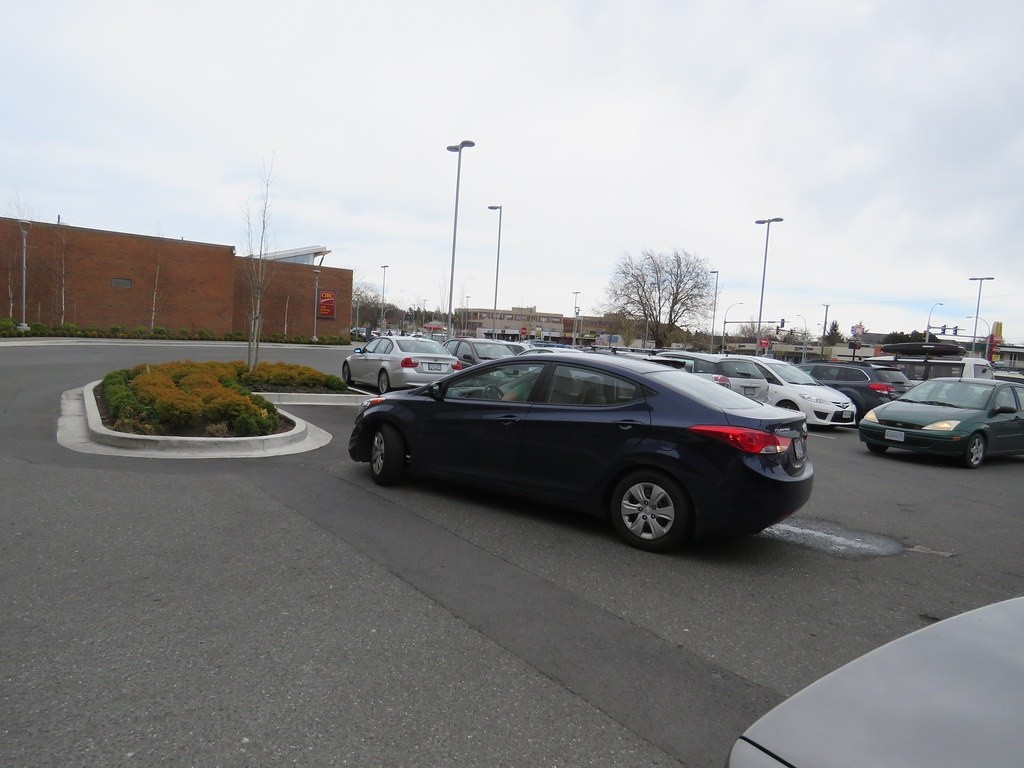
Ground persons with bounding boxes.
[401,330,404,336]
[387,330,393,336]
[499,382,534,401]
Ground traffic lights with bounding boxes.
[941,325,958,336]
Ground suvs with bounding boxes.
[796,359,914,429]
[992,366,1024,385]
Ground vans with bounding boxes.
[862,341,994,386]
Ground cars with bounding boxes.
[658,352,857,432]
[858,377,1024,469]
[347,353,814,551]
[342,327,586,395]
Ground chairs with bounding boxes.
[978,390,1002,408]
[549,368,575,404]
[577,376,607,405]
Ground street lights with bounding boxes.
[969,277,994,352]
[925,302,944,342]
[968,317,991,360]
[755,218,783,356]
[722,303,744,354]
[796,315,806,362]
[710,271,719,353]
[381,265,390,334]
[572,292,580,348]
[488,206,502,340]
[447,140,475,337]
[819,303,829,359]
